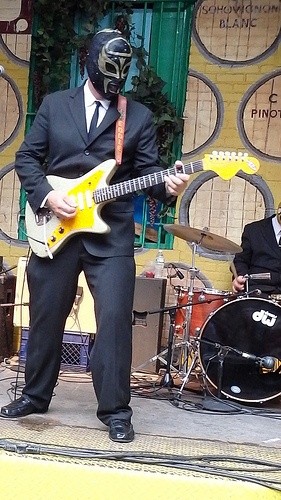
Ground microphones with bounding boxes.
[171,264,184,279]
[242,353,275,369]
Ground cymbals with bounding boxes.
[163,225,244,253]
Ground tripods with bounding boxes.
[130,241,250,406]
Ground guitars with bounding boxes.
[25,150,259,258]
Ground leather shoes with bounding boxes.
[108,419,134,443]
[0,396,43,418]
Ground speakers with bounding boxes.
[13,256,97,334]
[0,274,19,361]
[130,276,166,375]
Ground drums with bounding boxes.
[196,297,281,404]
[174,288,238,335]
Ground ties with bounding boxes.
[87,101,101,142]
[278,237,281,245]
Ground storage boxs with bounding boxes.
[19,328,90,373]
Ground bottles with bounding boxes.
[155,250,165,277]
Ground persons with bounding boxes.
[0,29,191,443]
[229,202,281,298]
